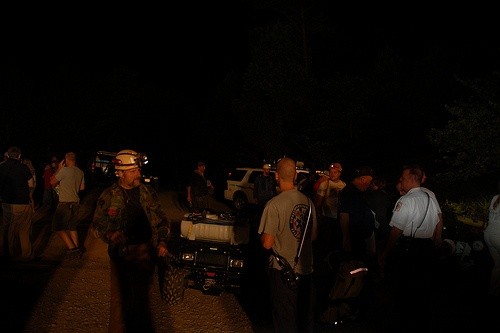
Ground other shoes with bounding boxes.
[78,244,85,256]
[70,248,81,269]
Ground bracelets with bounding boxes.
[158,239,168,246]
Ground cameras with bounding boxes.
[282,268,300,289]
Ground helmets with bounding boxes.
[263,164,271,169]
[114,150,145,170]
[329,163,343,172]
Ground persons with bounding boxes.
[90,150,170,333]
[0,148,21,264]
[484,194,500,315]
[19,156,59,215]
[380,163,440,332]
[186,159,214,213]
[294,168,331,200]
[50,152,85,257]
[258,158,318,333]
[340,165,378,322]
[386,176,407,319]
[312,159,346,257]
[253,164,278,231]
[0,146,40,264]
[362,173,390,251]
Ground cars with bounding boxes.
[215,168,324,213]
